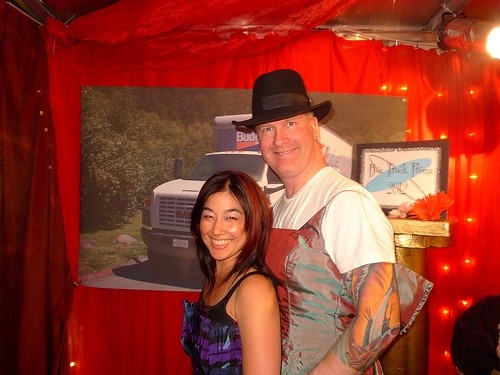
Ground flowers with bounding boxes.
[386,192,454,221]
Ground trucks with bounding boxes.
[140,113,353,274]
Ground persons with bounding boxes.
[182,170,286,375]
[231,68,401,375]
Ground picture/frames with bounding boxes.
[351,139,450,221]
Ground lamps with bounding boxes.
[437,5,500,54]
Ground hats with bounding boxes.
[232,69,332,128]
